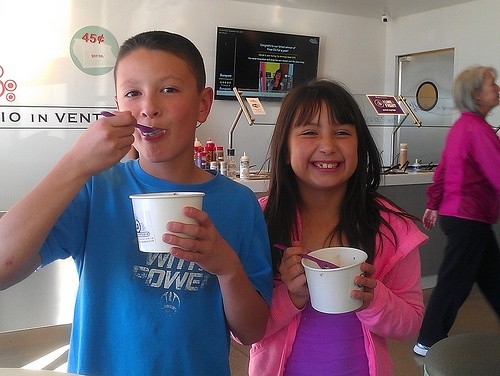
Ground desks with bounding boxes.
[234,165,435,193]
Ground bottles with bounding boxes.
[193,137,250,181]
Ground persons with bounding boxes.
[0,31,273,376]
[229,78,429,376]
[412,66,500,357]
[271,69,285,91]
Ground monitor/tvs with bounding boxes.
[212,27,320,101]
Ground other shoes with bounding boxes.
[413,342,430,358]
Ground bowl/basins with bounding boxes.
[300,247,368,314]
[128,192,205,254]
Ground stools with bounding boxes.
[425,332,500,376]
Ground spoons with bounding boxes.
[274,244,340,269]
[100,111,161,135]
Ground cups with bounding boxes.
[400,143,407,171]
